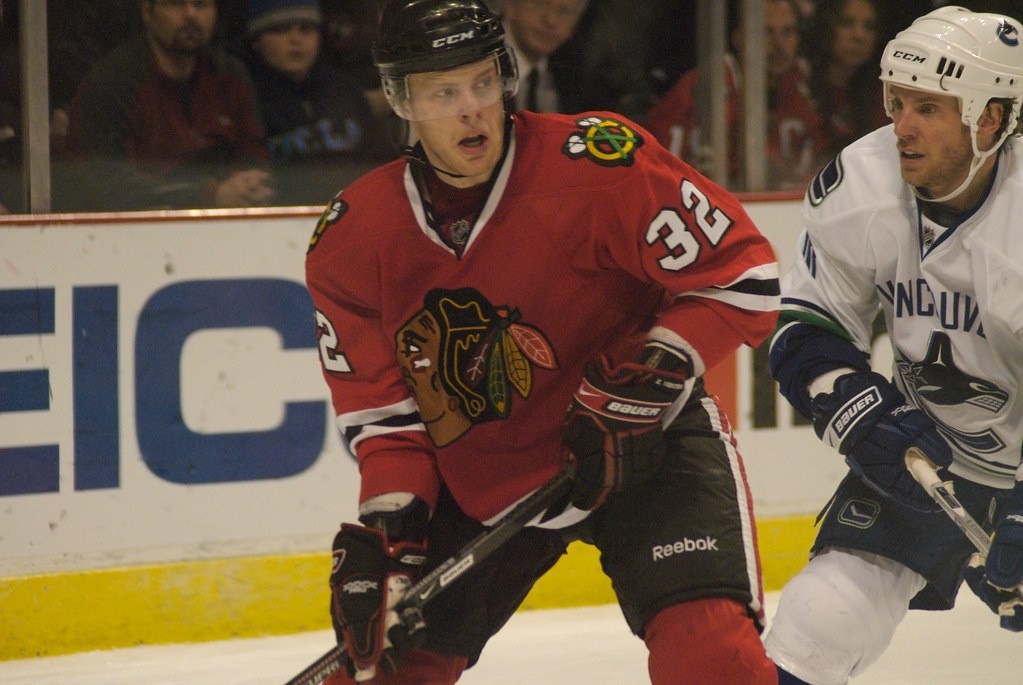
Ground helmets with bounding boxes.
[878,6,1023,127]
[373,0,519,119]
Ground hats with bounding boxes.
[238,0,322,43]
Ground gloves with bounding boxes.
[330,523,430,682]
[811,371,954,513]
[964,479,1023,633]
[536,354,685,530]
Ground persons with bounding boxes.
[305,0,779,684]
[52,0,890,215]
[762,5,1023,684]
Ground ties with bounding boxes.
[526,71,538,112]
